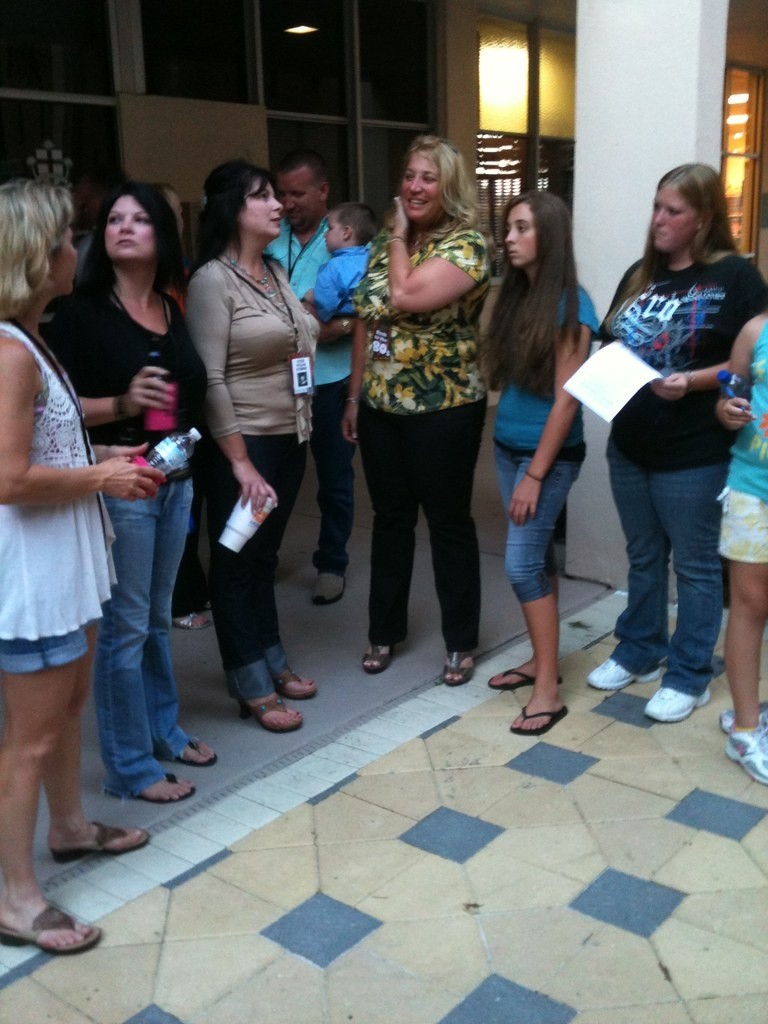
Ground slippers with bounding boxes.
[52,821,150,863]
[0,905,100,954]
[511,705,567,735]
[488,668,562,691]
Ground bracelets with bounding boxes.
[346,397,359,404]
[526,471,541,482]
[688,370,696,393]
[113,395,131,420]
[384,234,408,246]
[341,318,353,334]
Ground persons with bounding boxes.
[69,165,215,626]
[304,200,381,324]
[1,175,168,956]
[182,157,319,734]
[589,163,768,722]
[259,149,357,604]
[50,181,221,804]
[298,373,307,385]
[339,134,493,689]
[474,192,601,735]
[712,304,768,786]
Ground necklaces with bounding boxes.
[222,252,278,299]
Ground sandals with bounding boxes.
[172,612,212,629]
[176,738,217,766]
[443,650,475,686]
[135,772,195,802]
[362,644,392,673]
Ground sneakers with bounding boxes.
[726,713,768,784]
[721,701,768,733]
[588,636,669,689]
[644,683,710,722]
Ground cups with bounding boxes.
[218,493,274,553]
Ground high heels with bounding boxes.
[239,692,302,731]
[272,668,317,699]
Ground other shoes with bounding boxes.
[312,571,345,602]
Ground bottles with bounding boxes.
[716,370,751,403]
[140,340,178,429]
[144,427,202,477]
